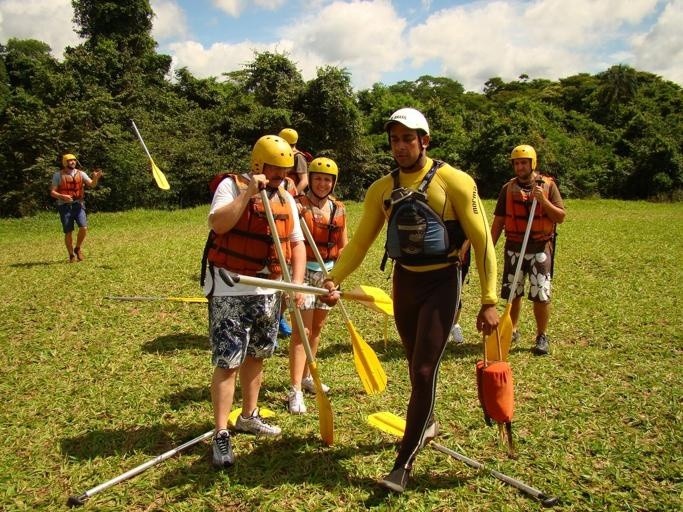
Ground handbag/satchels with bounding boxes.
[473,321,516,425]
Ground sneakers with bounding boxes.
[232,406,284,437]
[69,255,75,263]
[381,456,416,494]
[277,317,292,336]
[287,385,307,415]
[73,246,84,261]
[210,427,236,467]
[416,422,440,455]
[532,331,549,356]
[301,373,330,394]
[449,322,464,344]
[511,328,520,342]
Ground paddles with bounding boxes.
[67,406,275,507]
[261,188,334,447]
[300,215,388,397]
[231,273,394,316]
[104,296,208,303]
[368,412,557,507]
[483,179,544,362]
[131,121,171,190]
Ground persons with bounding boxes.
[491,145,566,355]
[51,154,102,262]
[319,108,499,493]
[200,129,348,471]
[448,243,472,344]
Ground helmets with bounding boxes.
[306,156,339,195]
[382,107,430,137]
[249,134,295,175]
[276,126,299,146]
[62,153,76,168]
[508,143,537,171]
